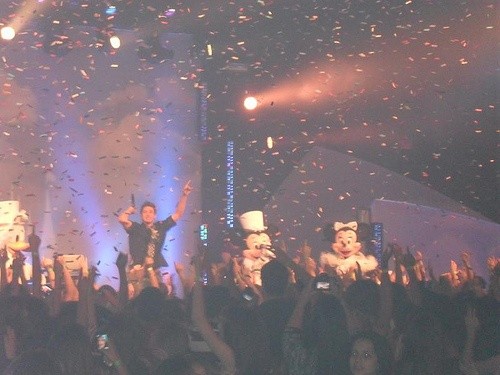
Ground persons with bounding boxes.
[0,211,499,374]
[116,179,193,290]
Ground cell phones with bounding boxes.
[94,331,109,351]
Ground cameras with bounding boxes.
[242,286,258,301]
[313,278,330,290]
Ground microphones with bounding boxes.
[131,193,135,208]
